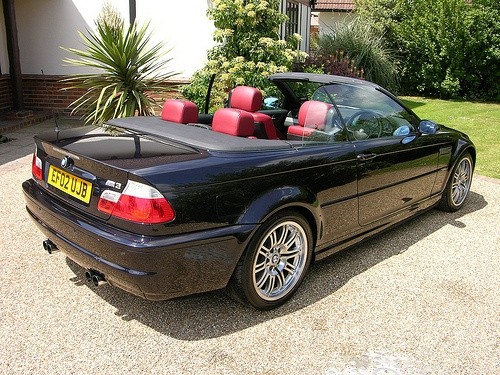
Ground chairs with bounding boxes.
[287,100,334,140]
[211,108,258,139]
[230,86,278,140]
[162,99,199,123]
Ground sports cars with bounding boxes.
[18,71,477,312]
